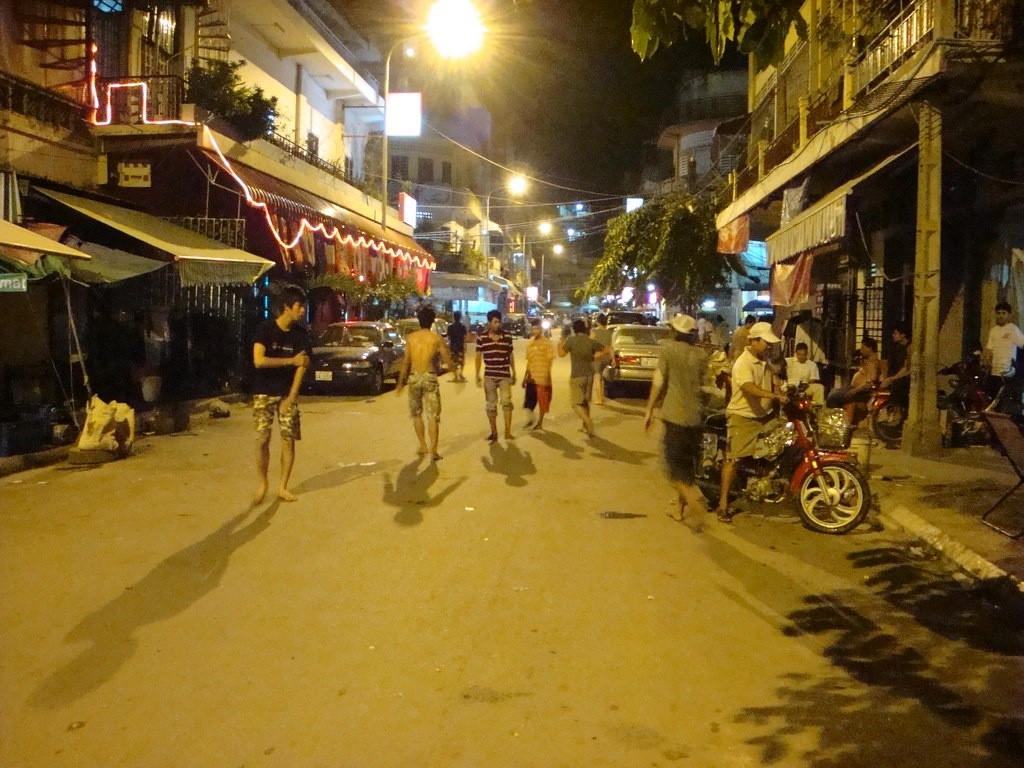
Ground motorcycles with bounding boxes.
[867,349,996,447]
[691,381,872,536]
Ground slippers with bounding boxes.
[717,507,732,522]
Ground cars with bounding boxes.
[599,323,674,397]
[541,310,601,339]
[307,321,406,398]
[396,317,454,365]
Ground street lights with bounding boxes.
[485,178,530,258]
[523,221,553,276]
[540,244,564,298]
[379,6,478,231]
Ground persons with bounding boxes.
[522,319,555,429]
[984,302,1024,400]
[698,312,756,359]
[644,314,708,521]
[396,306,448,460]
[784,343,824,400]
[782,302,830,384]
[715,321,790,523]
[475,309,516,440]
[827,338,880,408]
[558,320,606,438]
[447,311,467,381]
[252,285,316,502]
[572,312,592,330]
[881,322,912,407]
[590,313,616,405]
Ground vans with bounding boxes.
[605,311,648,326]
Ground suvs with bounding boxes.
[503,313,532,337]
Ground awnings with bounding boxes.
[763,143,919,265]
[193,149,437,271]
[33,186,275,287]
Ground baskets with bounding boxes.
[814,419,857,450]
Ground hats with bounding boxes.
[746,322,781,343]
[663,315,699,335]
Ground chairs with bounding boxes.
[980,411,1024,539]
[844,359,888,429]
